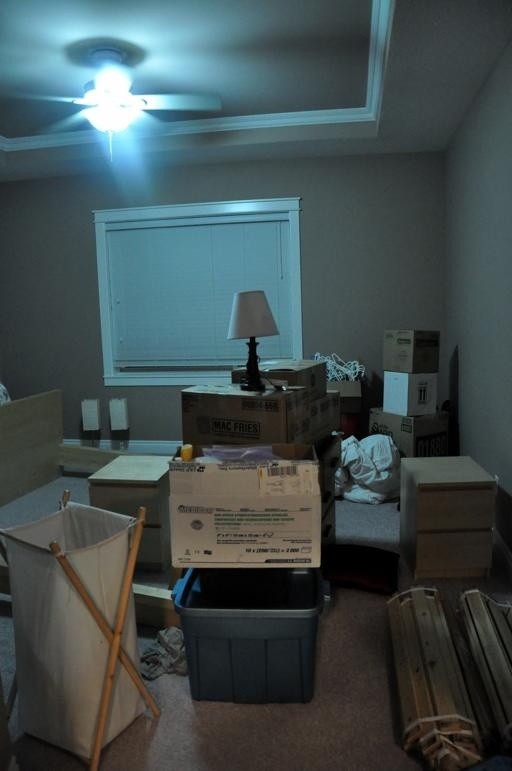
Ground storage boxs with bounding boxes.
[382,329,440,373]
[168,442,322,568]
[231,358,326,402]
[311,356,379,414]
[171,568,331,705]
[309,392,340,444]
[182,383,311,446]
[400,455,497,581]
[368,401,457,457]
[88,455,175,573]
[383,370,438,417]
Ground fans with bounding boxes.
[6,50,221,133]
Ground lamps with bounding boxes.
[227,291,280,392]
